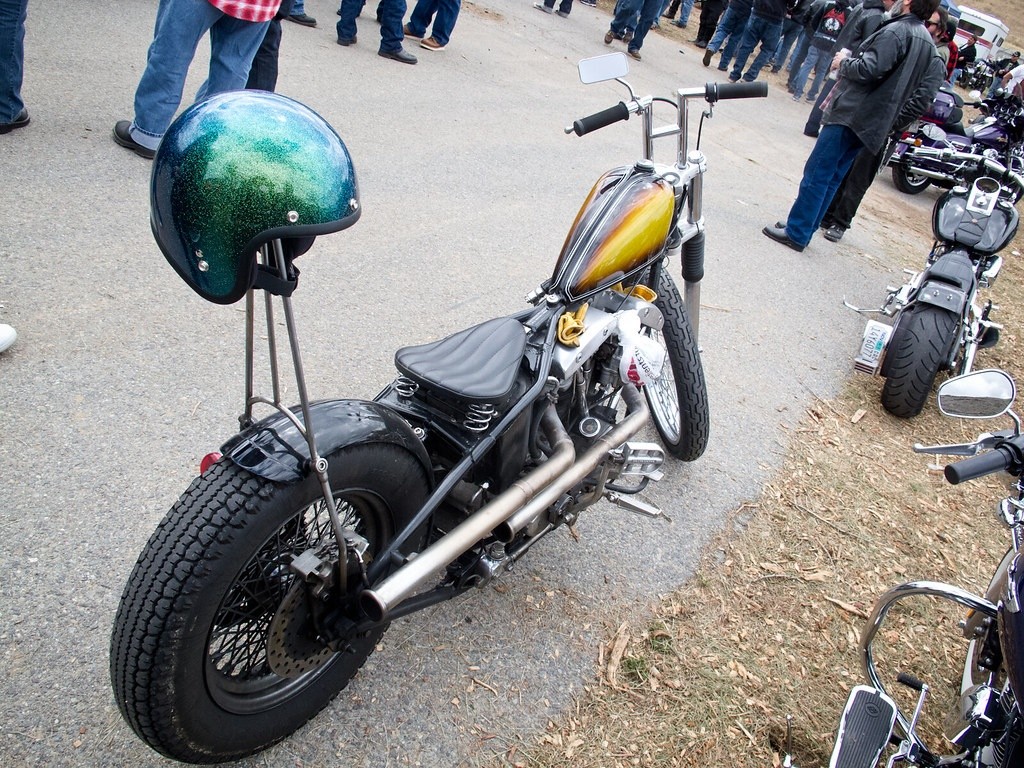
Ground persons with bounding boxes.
[0,0,31,134]
[761,0,941,253]
[535,0,1024,111]
[112,0,463,159]
[819,7,950,240]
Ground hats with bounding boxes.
[1012,51,1021,57]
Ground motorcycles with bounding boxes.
[843,122,1024,419]
[827,369,1024,768]
[108,49,769,766]
[959,57,994,95]
[889,79,1024,206]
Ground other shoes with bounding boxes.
[604,30,615,45]
[738,79,747,84]
[402,24,410,34]
[804,130,820,137]
[420,38,446,51]
[728,76,735,82]
[535,4,552,13]
[554,9,569,18]
[775,220,810,248]
[650,11,706,50]
[824,224,845,243]
[718,66,729,70]
[622,31,633,43]
[764,58,817,107]
[0,108,30,134]
[581,0,597,7]
[337,34,357,46]
[762,225,804,252]
[112,120,157,159]
[287,14,318,26]
[703,48,713,66]
[627,50,642,61]
[337,8,361,17]
[378,47,418,64]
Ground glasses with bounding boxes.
[924,20,939,28]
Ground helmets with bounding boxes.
[150,89,362,305]
